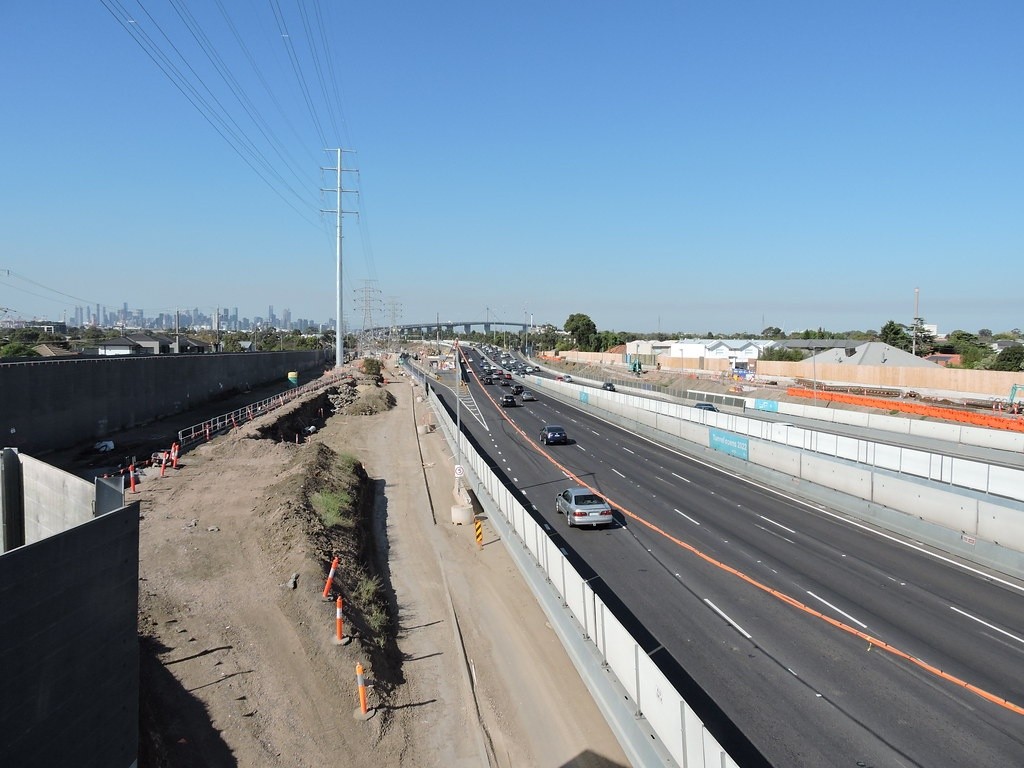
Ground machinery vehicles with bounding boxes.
[628,361,643,377]
[1001,384,1024,416]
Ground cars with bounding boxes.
[563,375,572,382]
[556,487,612,530]
[772,422,796,428]
[555,376,564,382]
[694,403,720,413]
[461,341,540,408]
[539,425,567,446]
[601,382,616,392]
